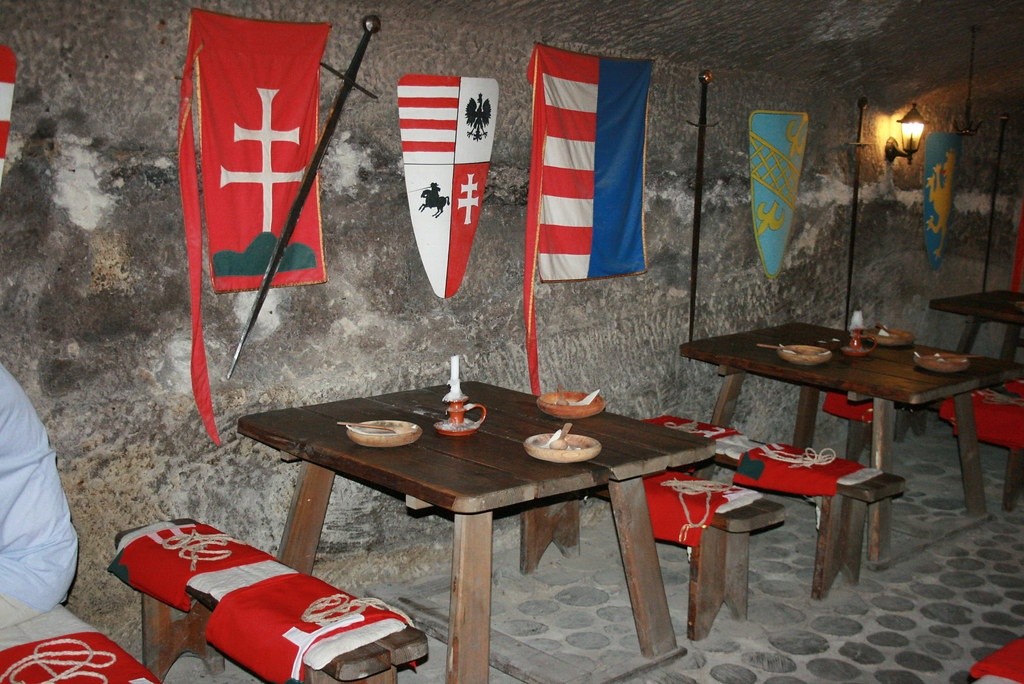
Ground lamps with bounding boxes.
[885,102,925,165]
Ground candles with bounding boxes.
[849,307,866,332]
[442,353,465,403]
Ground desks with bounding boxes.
[929,289,1024,365]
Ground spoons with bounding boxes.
[549,423,573,450]
[556,383,570,406]
[337,421,407,435]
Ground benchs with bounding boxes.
[637,416,906,601]
[107,518,430,684]
[521,469,786,640]
[990,377,1024,398]
[893,387,1024,512]
[0,603,164,684]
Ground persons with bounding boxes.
[0,360,80,614]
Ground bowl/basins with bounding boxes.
[346,420,423,448]
[863,327,914,346]
[536,392,605,418]
[914,352,969,373]
[522,433,602,462]
[777,345,832,365]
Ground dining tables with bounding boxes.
[238,380,718,684]
[680,321,1024,561]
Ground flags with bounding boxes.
[178,9,332,446]
[523,42,655,397]
